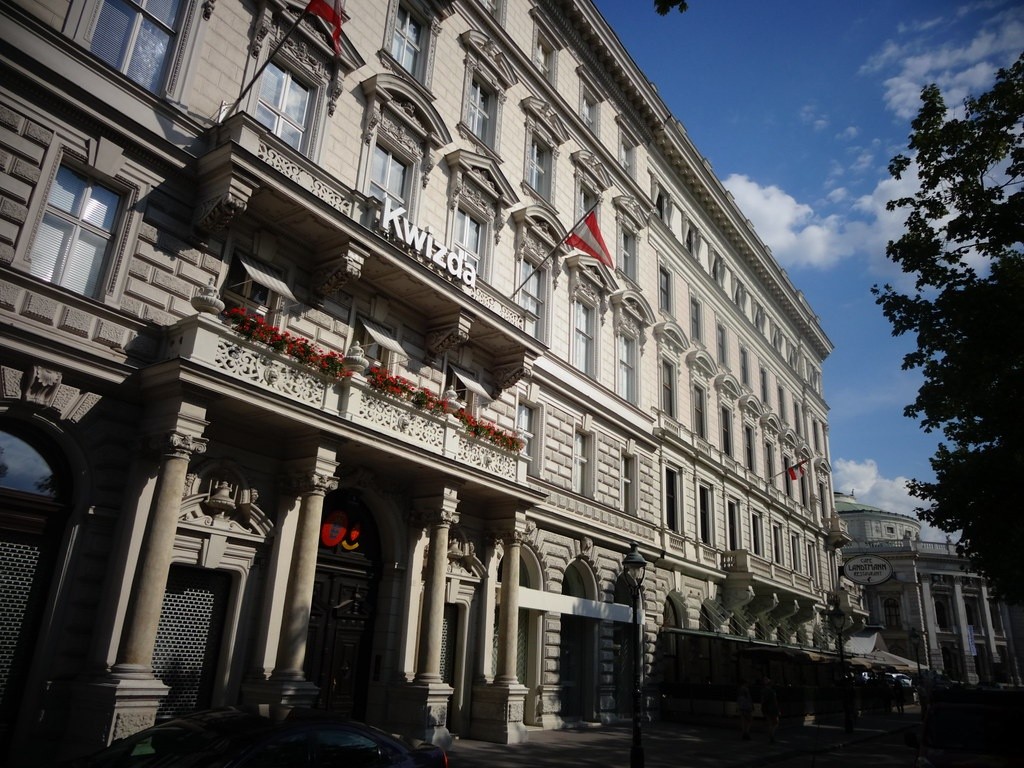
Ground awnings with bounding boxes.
[665,592,844,656]
[845,650,929,671]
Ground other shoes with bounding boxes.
[768,736,776,743]
[742,733,751,740]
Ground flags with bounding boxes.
[565,211,614,270]
[788,465,805,480]
[308,0,344,58]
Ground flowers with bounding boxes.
[221,308,526,453]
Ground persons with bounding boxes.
[762,678,781,742]
[736,679,754,740]
[880,684,905,715]
